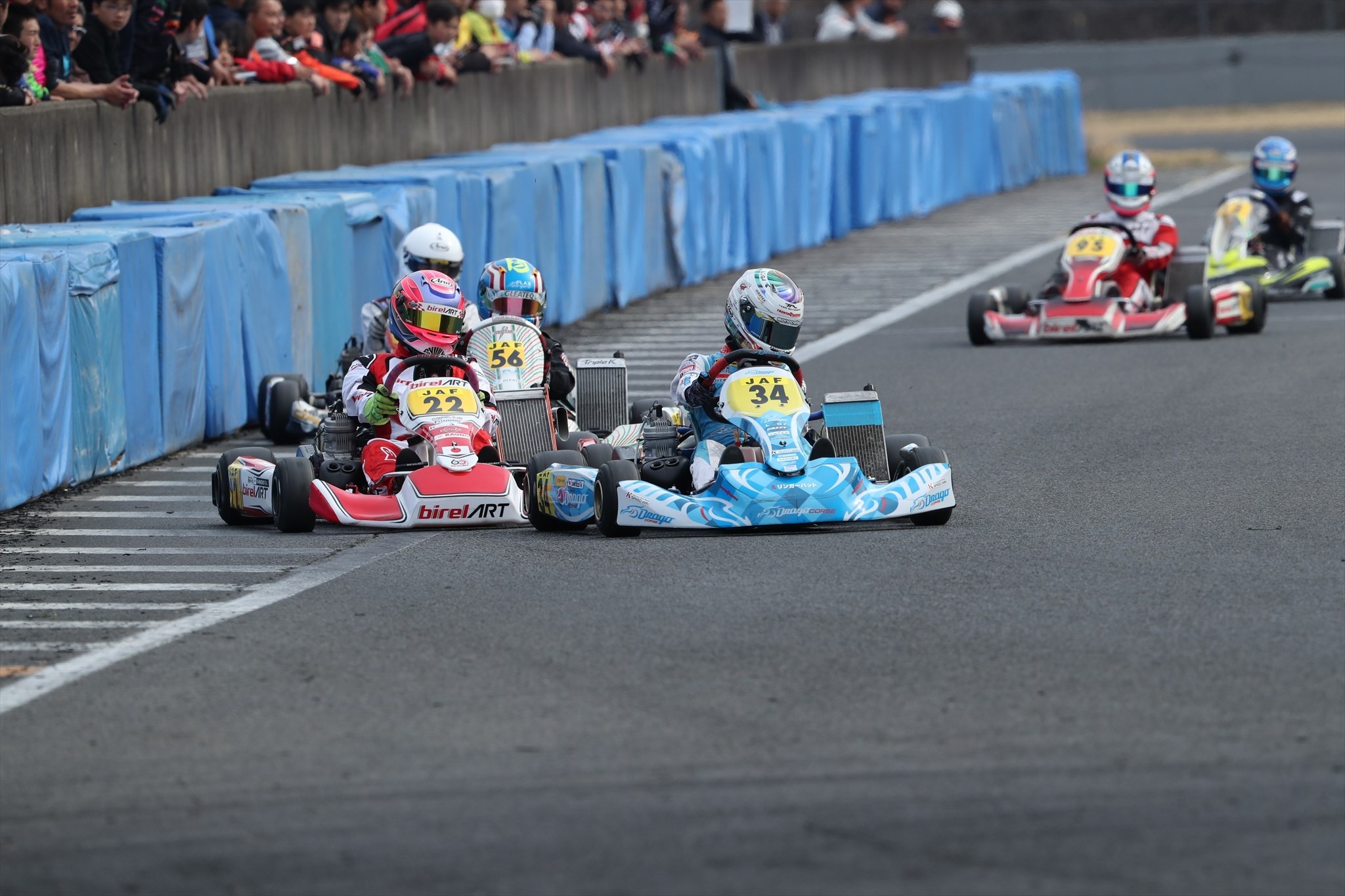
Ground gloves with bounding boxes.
[476,390,486,408]
[369,314,388,350]
[372,385,399,420]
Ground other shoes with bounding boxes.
[395,449,425,490]
[718,445,746,467]
[808,437,837,461]
[477,445,502,466]
[1127,247,1144,263]
[685,372,714,407]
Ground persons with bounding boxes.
[1250,136,1314,268]
[0,0,966,106]
[671,268,836,491]
[341,224,576,495]
[1042,150,1178,313]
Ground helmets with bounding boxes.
[476,257,547,331]
[396,222,464,286]
[1252,136,1298,191]
[389,269,466,355]
[1105,148,1156,216]
[724,267,804,367]
